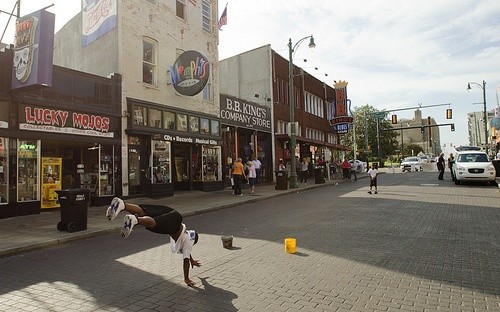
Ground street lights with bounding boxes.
[466,80,489,154]
[287,34,317,189]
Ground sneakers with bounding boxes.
[120,214,138,238]
[106,197,125,221]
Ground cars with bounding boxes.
[400,156,436,172]
[347,160,368,169]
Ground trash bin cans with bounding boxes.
[54,187,91,233]
[274,170,288,190]
[313,164,325,184]
[492,160,500,177]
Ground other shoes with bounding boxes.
[367,191,377,194]
[232,191,255,196]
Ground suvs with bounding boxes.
[450,145,497,185]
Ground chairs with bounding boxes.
[460,155,469,162]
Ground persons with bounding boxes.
[436,153,441,172]
[245,161,256,194]
[229,159,237,195]
[437,153,445,180]
[448,153,454,178]
[367,164,378,194]
[287,155,369,185]
[232,158,247,196]
[243,158,250,186]
[106,197,202,287]
[251,156,262,188]
[279,160,286,172]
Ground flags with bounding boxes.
[217,6,227,31]
